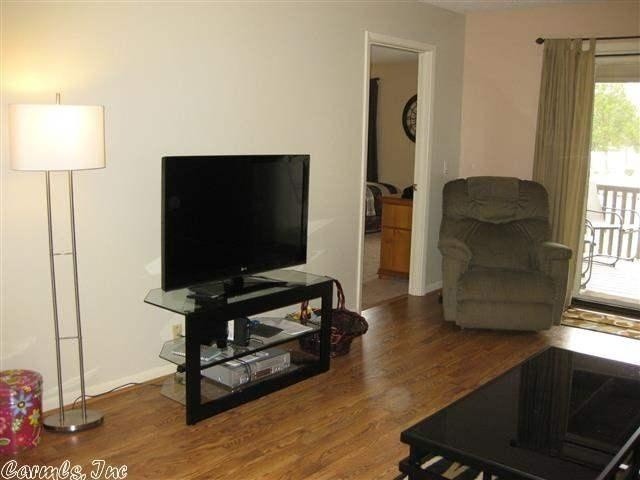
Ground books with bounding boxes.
[172,342,222,362]
[275,320,313,337]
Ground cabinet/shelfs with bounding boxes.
[378,196,412,280]
[142,269,335,427]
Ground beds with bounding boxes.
[367,182,399,232]
[396,345,639,479]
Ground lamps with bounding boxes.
[9,94,106,433]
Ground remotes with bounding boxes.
[187,293,227,303]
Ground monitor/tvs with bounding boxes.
[162,155,310,298]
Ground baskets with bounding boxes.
[285,276,368,358]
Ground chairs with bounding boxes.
[578,181,639,291]
[440,177,571,333]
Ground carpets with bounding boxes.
[560,308,640,341]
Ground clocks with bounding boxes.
[401,93,417,143]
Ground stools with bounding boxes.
[0,369,45,456]
